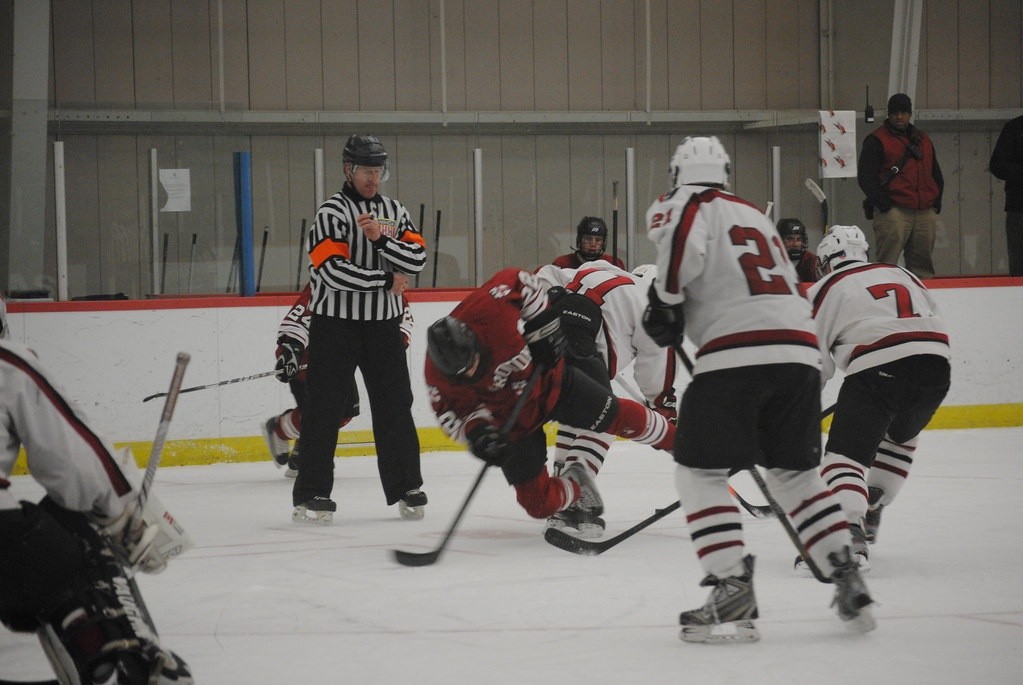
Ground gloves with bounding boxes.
[522,310,566,375]
[470,424,516,468]
[642,277,684,347]
[274,342,303,384]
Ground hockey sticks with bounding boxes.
[226,238,238,293]
[613,373,776,519]
[256,227,269,292]
[673,341,831,584]
[805,178,828,233]
[764,200,774,217]
[143,364,308,403]
[415,204,424,288]
[160,233,169,294]
[123,352,191,544]
[189,233,197,293]
[545,402,839,556]
[612,182,618,266]
[296,219,306,291]
[432,210,441,288]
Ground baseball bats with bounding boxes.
[395,362,545,566]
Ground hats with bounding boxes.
[886,91,914,113]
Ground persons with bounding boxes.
[793,225,951,578]
[292,134,426,525]
[858,93,945,278]
[776,218,817,283]
[424,266,676,518]
[989,115,1023,276]
[645,134,877,642]
[536,265,679,536]
[552,217,625,270]
[0,299,193,685]
[260,281,413,479]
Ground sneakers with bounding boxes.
[399,489,428,520]
[261,408,290,470]
[568,458,605,518]
[294,494,338,526]
[677,554,761,643]
[820,543,877,633]
[865,483,882,544]
[794,520,871,578]
[285,435,302,476]
[544,507,607,539]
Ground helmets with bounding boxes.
[669,135,730,190]
[776,217,809,260]
[575,217,610,260]
[427,318,486,387]
[342,133,387,168]
[816,223,871,275]
[632,263,656,281]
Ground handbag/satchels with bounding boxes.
[862,198,874,220]
